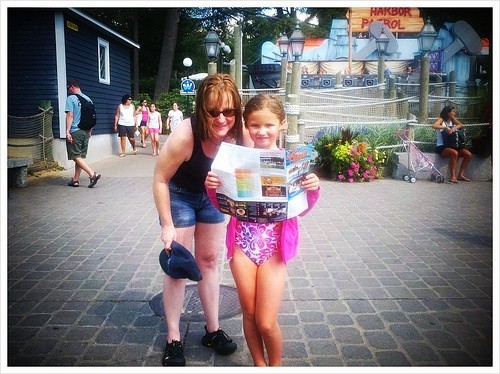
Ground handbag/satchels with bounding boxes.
[440,126,466,151]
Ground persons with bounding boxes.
[147,103,163,155]
[433,107,472,183]
[115,94,139,157]
[153,74,253,367]
[139,99,151,148]
[133,105,143,136]
[65,79,101,188]
[204,93,320,367]
[167,102,184,134]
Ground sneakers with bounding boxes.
[202,325,237,355]
[162,339,186,367]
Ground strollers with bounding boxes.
[396,128,447,184]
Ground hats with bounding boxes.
[159,240,204,281]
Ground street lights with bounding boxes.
[183,57,193,105]
[204,31,221,76]
[416,15,439,125]
[220,42,232,74]
[281,21,306,151]
[375,27,390,118]
[278,32,290,101]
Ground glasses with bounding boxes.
[207,105,238,118]
[128,99,132,100]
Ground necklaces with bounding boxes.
[207,135,227,145]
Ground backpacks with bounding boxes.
[74,94,97,130]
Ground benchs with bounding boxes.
[392,149,493,180]
[7,156,34,188]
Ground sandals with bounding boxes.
[67,178,79,187]
[88,172,101,188]
